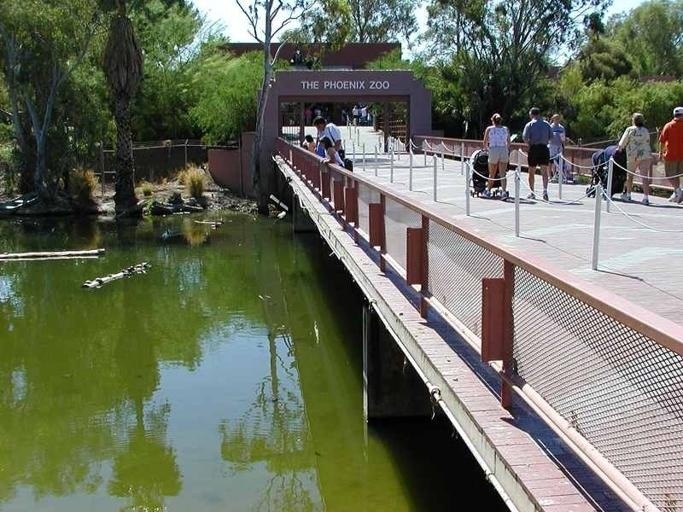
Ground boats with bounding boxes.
[0,189,38,214]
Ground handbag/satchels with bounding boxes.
[337,149,345,159]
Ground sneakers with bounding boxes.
[620,193,631,201]
[499,191,508,201]
[667,188,683,205]
[639,198,650,206]
[527,190,548,202]
[480,188,490,199]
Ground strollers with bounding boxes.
[466,148,508,199]
[587,146,627,199]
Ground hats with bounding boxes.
[672,106,683,116]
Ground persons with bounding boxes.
[549,114,569,180]
[616,113,653,205]
[522,107,554,202]
[300,104,373,167]
[482,113,511,197]
[655,106,683,203]
